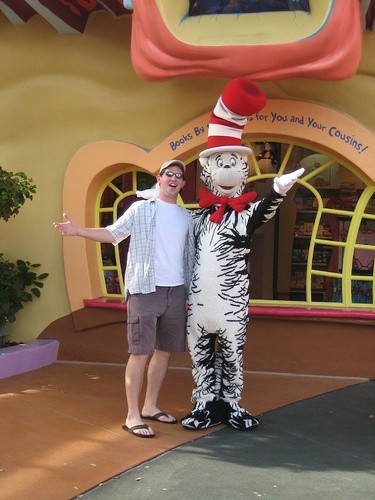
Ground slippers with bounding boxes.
[122,424,154,438]
[140,411,177,424]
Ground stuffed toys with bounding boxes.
[135,78,306,431]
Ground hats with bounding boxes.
[159,159,185,174]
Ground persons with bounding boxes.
[53,160,196,438]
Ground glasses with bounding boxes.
[162,172,183,178]
